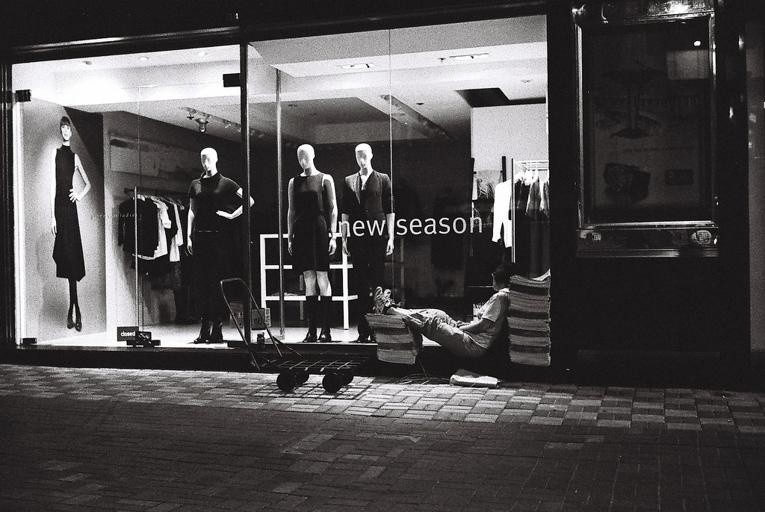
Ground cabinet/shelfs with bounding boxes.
[259,232,359,330]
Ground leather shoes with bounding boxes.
[66,312,81,332]
[349,337,376,344]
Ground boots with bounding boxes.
[205,318,224,343]
[193,316,210,344]
[302,295,318,343]
[320,295,333,341]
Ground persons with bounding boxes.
[371,261,523,362]
[286,143,339,343]
[340,142,396,343]
[186,146,256,345]
[49,115,93,332]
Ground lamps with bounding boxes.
[195,115,210,134]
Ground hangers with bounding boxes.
[515,159,549,184]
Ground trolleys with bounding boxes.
[219,277,365,393]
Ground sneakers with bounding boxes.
[372,286,393,314]
